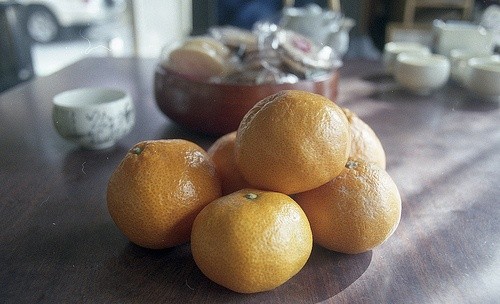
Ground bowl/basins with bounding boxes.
[152,52,341,139]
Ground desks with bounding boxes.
[0,55,500,304]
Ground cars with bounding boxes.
[0,0,127,44]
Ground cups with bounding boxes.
[384,23,436,49]
[450,49,487,90]
[433,20,486,58]
[52,88,136,150]
[395,53,450,96]
[468,58,500,103]
[382,40,432,77]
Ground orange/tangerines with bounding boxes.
[107,89,402,292]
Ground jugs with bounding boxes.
[278,4,355,60]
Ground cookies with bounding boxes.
[167,25,343,85]
